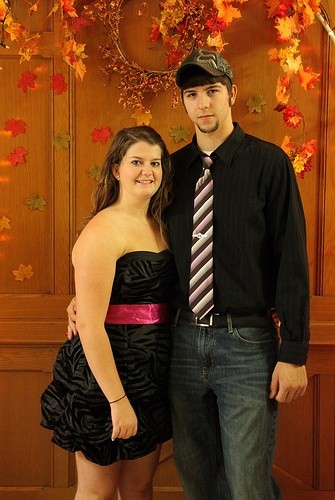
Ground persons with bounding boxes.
[65,48,312,500]
[40,126,179,500]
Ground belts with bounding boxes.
[178,310,270,328]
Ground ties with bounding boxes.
[188,156,214,320]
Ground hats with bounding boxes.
[176,50,233,86]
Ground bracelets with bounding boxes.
[109,394,126,404]
